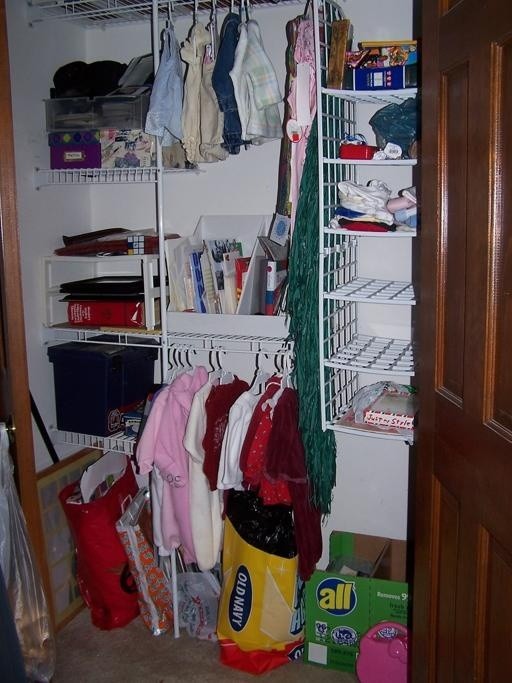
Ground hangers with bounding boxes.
[162,1,174,42]
[229,1,253,35]
[163,339,297,397]
[193,0,198,27]
[207,0,217,35]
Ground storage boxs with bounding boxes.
[305,530,409,674]
[100,127,152,169]
[48,129,101,177]
[47,335,159,437]
[43,95,149,132]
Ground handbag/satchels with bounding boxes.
[217,514,304,677]
[115,488,174,636]
[57,456,139,630]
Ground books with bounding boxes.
[258,258,289,315]
[170,239,251,315]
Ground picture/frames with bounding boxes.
[35,442,104,634]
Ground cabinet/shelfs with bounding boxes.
[24,0,308,457]
[313,1,419,444]
